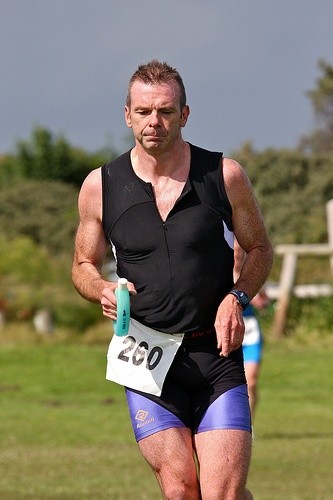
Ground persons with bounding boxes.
[70,59,275,500]
[231,232,272,442]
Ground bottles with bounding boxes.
[112,278,130,336]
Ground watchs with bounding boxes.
[229,289,250,311]
